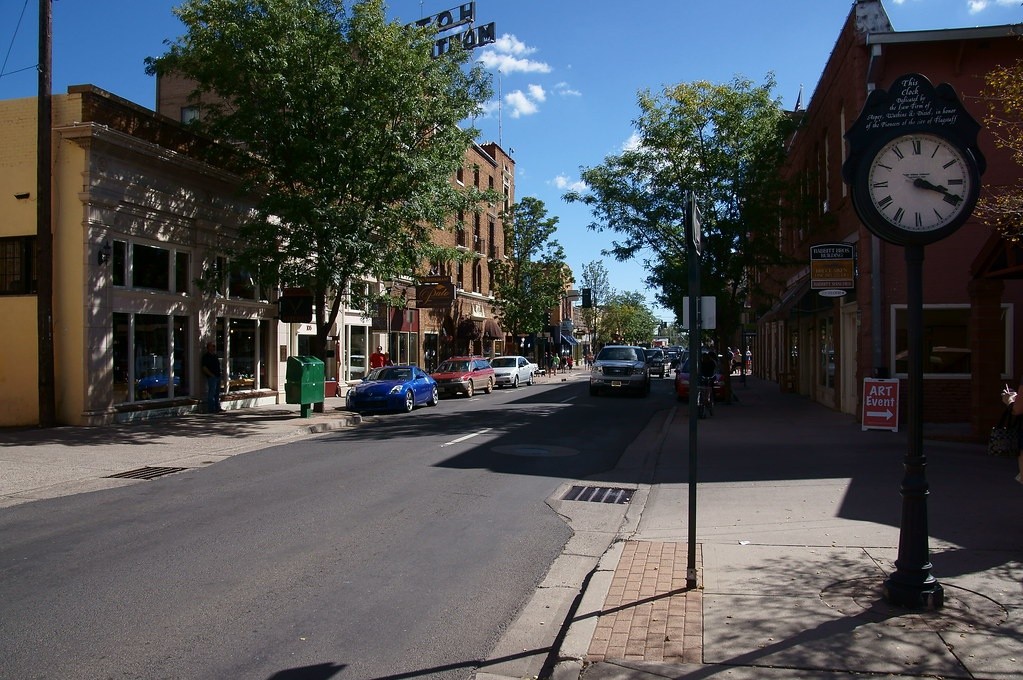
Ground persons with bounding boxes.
[551,353,560,376]
[383,352,394,367]
[548,352,553,378]
[728,347,734,372]
[560,354,567,373]
[735,349,742,374]
[369,346,385,369]
[744,345,752,373]
[201,343,227,413]
[587,353,594,370]
[1002,374,1023,484]
[567,355,573,372]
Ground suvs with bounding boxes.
[588,345,654,397]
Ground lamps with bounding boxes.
[99,241,110,266]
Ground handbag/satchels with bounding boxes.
[984,402,1021,457]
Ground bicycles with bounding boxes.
[697,372,718,420]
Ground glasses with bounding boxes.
[209,345,215,348]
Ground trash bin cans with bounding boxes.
[284,354,326,404]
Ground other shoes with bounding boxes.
[218,409,226,412]
[209,410,219,413]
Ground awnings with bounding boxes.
[561,334,579,346]
[760,276,832,322]
[485,320,503,338]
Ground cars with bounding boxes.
[489,356,538,389]
[137,371,182,401]
[645,344,712,380]
[345,367,439,413]
[428,355,497,398]
[673,356,727,401]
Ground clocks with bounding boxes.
[868,133,973,233]
